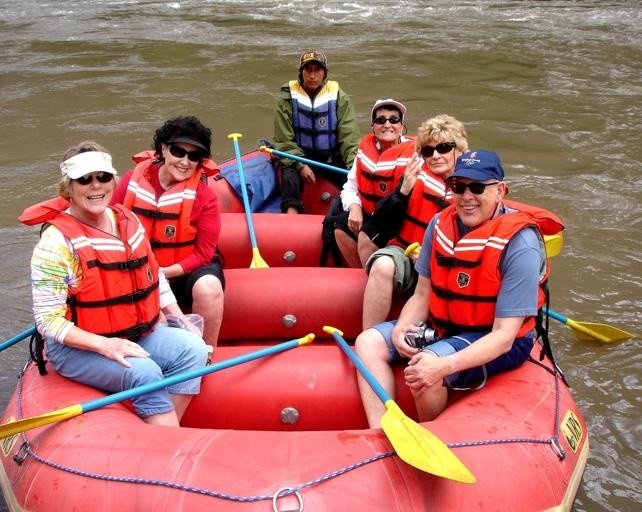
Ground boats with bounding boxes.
[0,141,589,512]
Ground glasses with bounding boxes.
[374,116,400,125]
[450,182,498,194]
[77,173,114,185]
[421,142,456,157]
[169,145,201,163]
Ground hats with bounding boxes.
[301,51,327,68]
[444,150,504,182]
[61,151,118,179]
[371,99,406,124]
[164,136,207,150]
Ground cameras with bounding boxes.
[405,320,439,349]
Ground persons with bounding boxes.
[107,116,225,384]
[354,149,550,428]
[30,141,208,428]
[362,113,469,332]
[272,49,361,214]
[333,99,419,269]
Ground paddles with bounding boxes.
[322,325,477,484]
[0,332,316,440]
[227,133,270,269]
[404,241,633,344]
